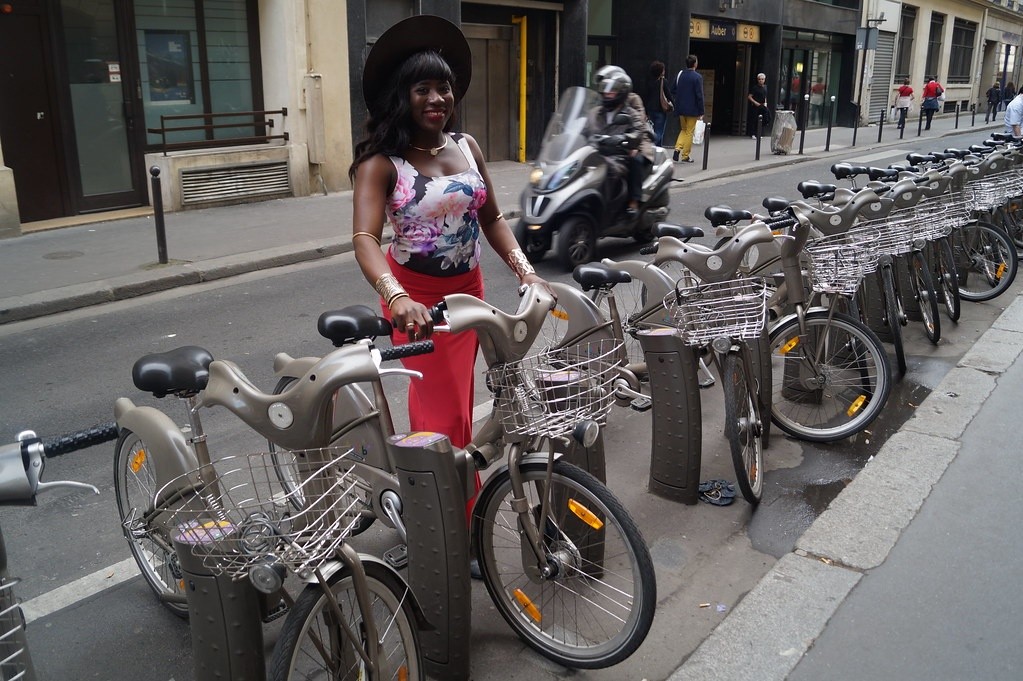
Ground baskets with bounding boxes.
[802,170,1023,294]
[663,276,766,347]
[154,446,362,581]
[482,338,623,439]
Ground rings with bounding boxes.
[406,323,415,326]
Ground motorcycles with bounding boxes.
[512,85,676,270]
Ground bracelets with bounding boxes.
[376,272,410,310]
[509,249,535,280]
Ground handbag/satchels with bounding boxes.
[936,84,941,97]
[660,76,674,112]
[692,117,706,145]
[891,108,898,121]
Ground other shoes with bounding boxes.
[683,157,693,163]
[627,206,638,216]
[471,560,483,579]
[752,135,756,139]
[673,150,679,161]
[897,125,902,129]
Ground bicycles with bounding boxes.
[518,129,1023,502]
[269,284,658,671]
[0,422,123,679]
[111,340,433,681]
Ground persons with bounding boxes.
[748,72,767,140]
[985,81,1001,124]
[1004,84,1023,135]
[922,77,944,130]
[350,15,555,578]
[895,78,915,129]
[585,64,655,219]
[811,76,826,125]
[643,61,674,146]
[672,54,704,163]
[1002,82,1016,109]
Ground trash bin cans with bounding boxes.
[771,110,797,154]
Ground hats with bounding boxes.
[363,14,472,115]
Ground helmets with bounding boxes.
[601,72,633,106]
[597,66,625,81]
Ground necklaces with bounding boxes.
[409,135,448,156]
